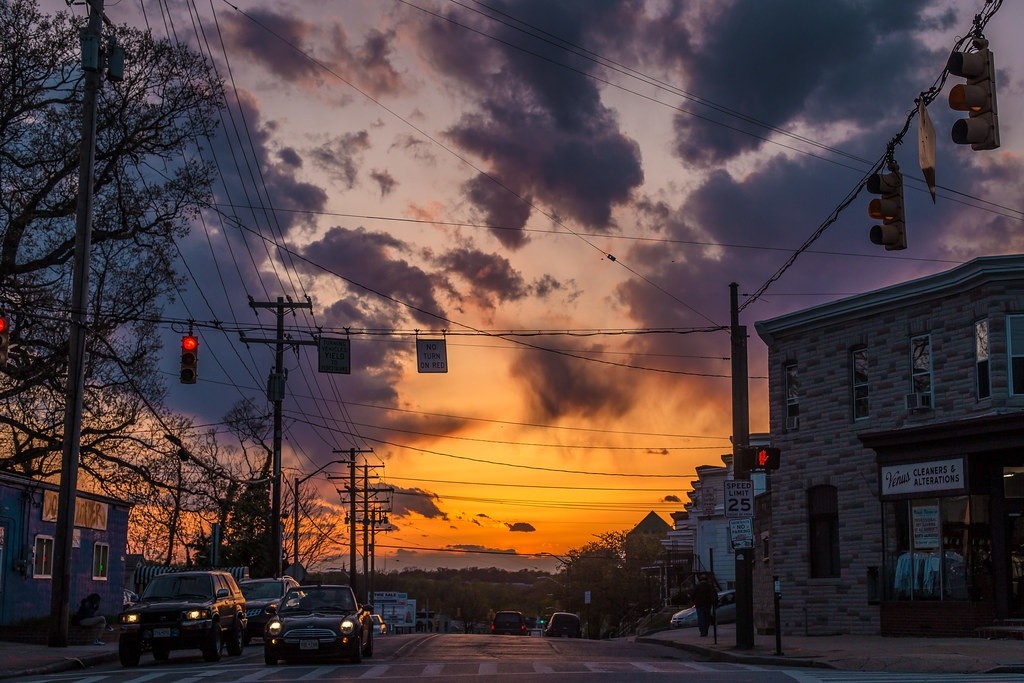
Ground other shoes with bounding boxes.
[106,626,114,632]
[93,638,105,645]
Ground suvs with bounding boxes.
[370,615,387,635]
[235,576,305,641]
[118,571,247,664]
[670,590,737,629]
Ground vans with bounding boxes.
[545,612,583,639]
[492,610,528,636]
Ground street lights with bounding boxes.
[536,551,571,610]
[295,460,352,580]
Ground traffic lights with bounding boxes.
[948,49,1000,152]
[1,316,10,365]
[178,336,198,384]
[865,172,907,251]
[755,447,784,470]
[537,621,544,623]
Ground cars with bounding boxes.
[122,588,138,609]
[264,586,373,663]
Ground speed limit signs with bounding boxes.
[724,481,754,518]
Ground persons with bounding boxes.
[71,593,114,645]
[693,574,718,637]
[687,593,724,608]
